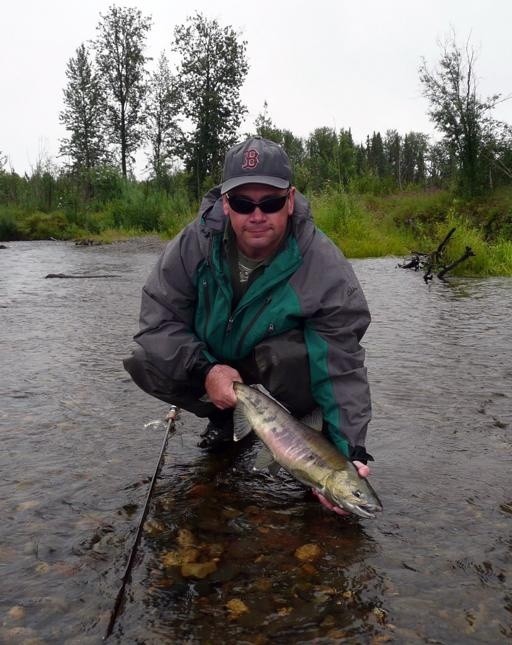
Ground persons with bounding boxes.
[125,134,372,518]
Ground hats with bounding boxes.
[220,136,292,196]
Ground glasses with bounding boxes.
[226,189,290,214]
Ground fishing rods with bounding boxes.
[101,404,177,641]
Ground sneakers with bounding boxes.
[198,422,227,448]
